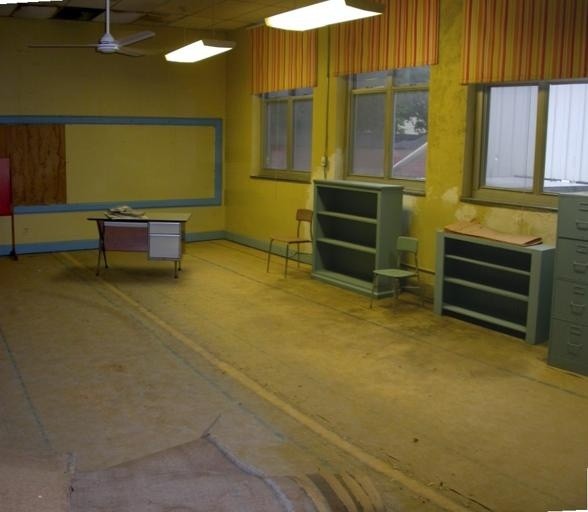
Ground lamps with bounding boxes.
[264,0,384,32]
[164,0,237,64]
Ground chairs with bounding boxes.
[370,236,425,314]
[267,209,313,278]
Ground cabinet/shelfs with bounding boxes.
[433,230,555,345]
[311,179,405,300]
[547,190,588,377]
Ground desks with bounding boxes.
[86,213,192,279]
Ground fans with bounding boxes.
[28,0,156,57]
